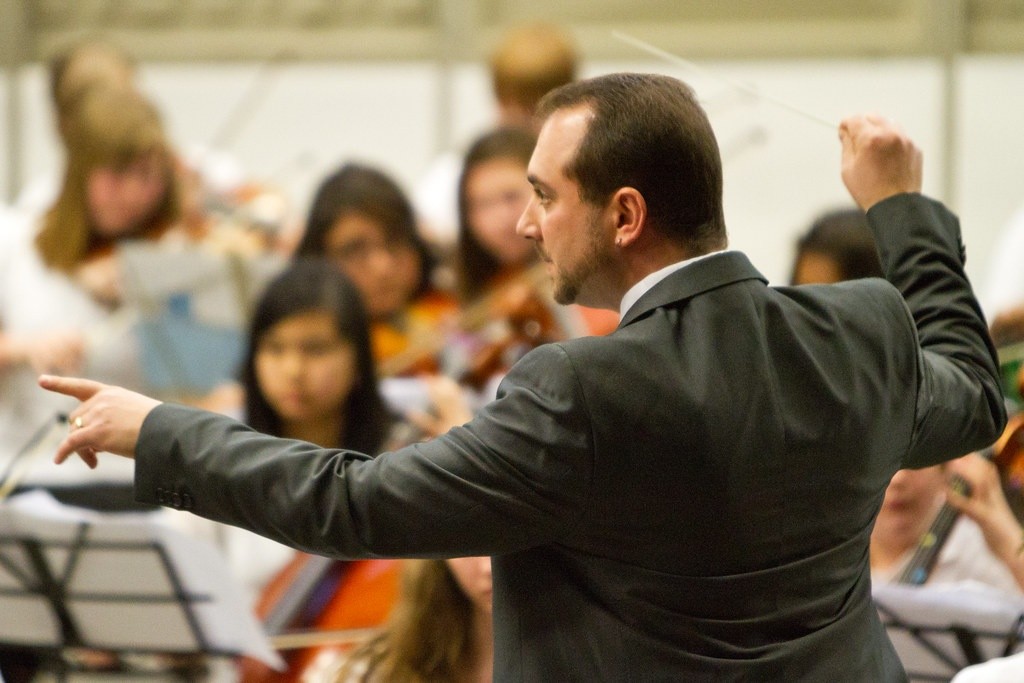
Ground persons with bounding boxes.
[780,206,1024,682]
[37,25,625,681]
[39,73,1009,683]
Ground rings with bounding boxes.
[73,415,83,429]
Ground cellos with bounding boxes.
[872,410,1023,585]
[225,285,549,683]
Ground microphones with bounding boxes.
[0,412,69,490]
